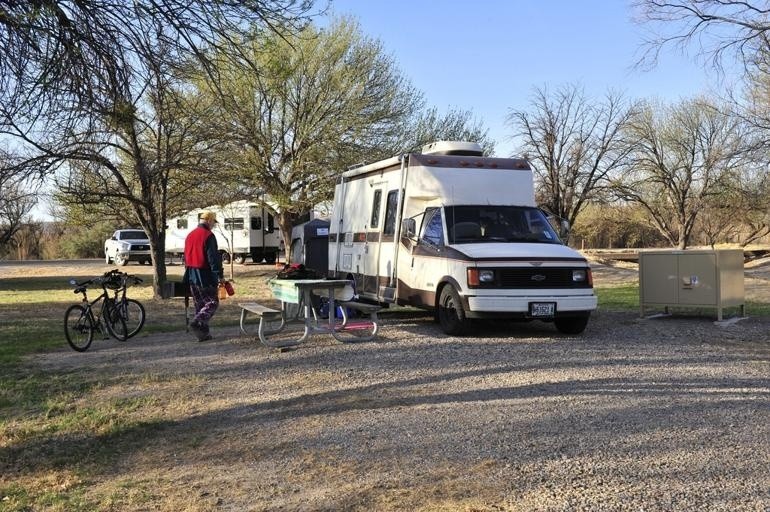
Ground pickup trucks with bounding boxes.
[105,229,152,266]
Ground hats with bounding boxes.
[200,212,219,223]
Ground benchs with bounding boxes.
[337,301,382,342]
[238,302,281,351]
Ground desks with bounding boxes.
[268,278,353,349]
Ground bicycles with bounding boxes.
[109,271,145,339]
[64,269,128,352]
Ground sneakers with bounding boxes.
[198,335,212,342]
[188,320,204,339]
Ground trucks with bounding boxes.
[165,200,280,264]
[328,141,597,335]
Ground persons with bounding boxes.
[184,212,226,341]
